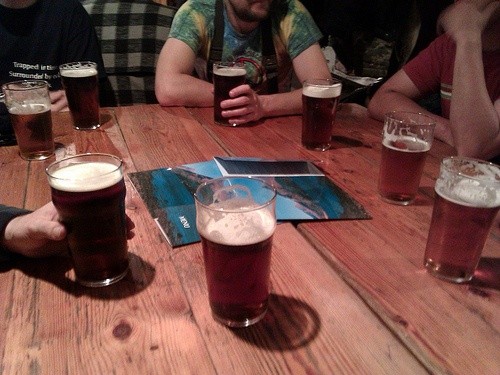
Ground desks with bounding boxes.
[0,102,500,375]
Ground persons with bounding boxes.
[369,0,500,162]
[0,199,136,259]
[0,0,347,126]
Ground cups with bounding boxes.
[0,93,17,146]
[212,61,248,126]
[194,176,277,327]
[301,79,342,151]
[59,61,101,130]
[423,156,500,283]
[2,80,55,161]
[379,110,437,205]
[45,152,129,288]
